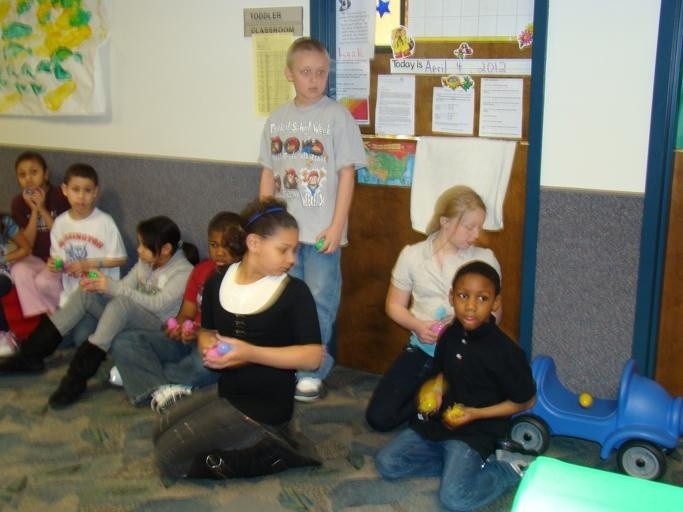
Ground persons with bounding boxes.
[1,150,129,376]
[261,37,367,403]
[111,211,244,414]
[361,184,504,433]
[48,217,200,410]
[153,196,323,480]
[374,260,540,511]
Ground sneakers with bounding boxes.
[0,330,17,357]
[293,377,322,403]
[154,384,193,413]
[495,437,538,467]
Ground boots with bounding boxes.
[48,338,107,410]
[0,316,62,375]
[188,430,322,479]
